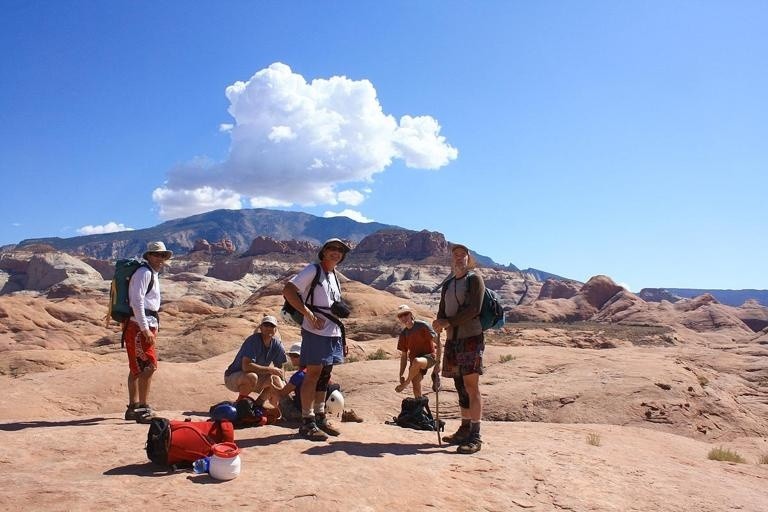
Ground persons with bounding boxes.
[282,236,351,442]
[124,241,173,424]
[394,303,442,398]
[430,243,485,454]
[224,316,287,406]
[262,341,331,422]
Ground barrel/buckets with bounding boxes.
[207,441,241,481]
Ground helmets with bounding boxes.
[325,390,345,412]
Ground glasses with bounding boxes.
[150,252,165,257]
[328,246,344,252]
[289,353,299,358]
[398,312,410,318]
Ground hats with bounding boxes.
[285,342,302,355]
[318,238,351,264]
[261,316,277,327]
[142,241,173,259]
[397,305,409,315]
[452,244,470,255]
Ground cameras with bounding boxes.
[331,301,350,318]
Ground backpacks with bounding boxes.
[325,383,340,399]
[281,264,321,326]
[107,259,153,322]
[466,271,505,331]
[385,396,445,431]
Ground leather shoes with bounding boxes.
[134,405,156,424]
[125,406,135,420]
[342,410,363,422]
[316,412,340,436]
[442,426,469,444]
[299,416,329,441]
[457,432,481,454]
[431,371,441,392]
